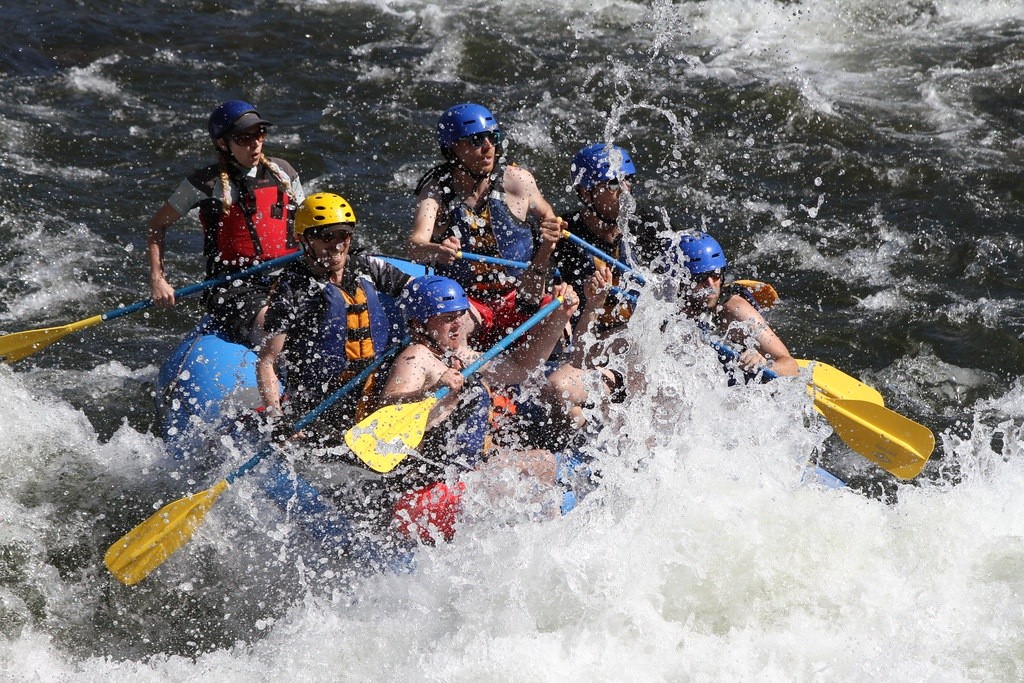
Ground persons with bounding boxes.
[255,103,801,539]
[149,99,306,352]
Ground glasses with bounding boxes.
[688,268,723,283]
[597,175,636,192]
[458,133,500,148]
[226,127,267,146]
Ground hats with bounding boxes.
[224,112,273,137]
[310,224,354,236]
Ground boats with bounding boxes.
[157,255,847,573]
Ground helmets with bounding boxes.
[399,276,470,323]
[208,100,261,149]
[664,231,728,278]
[437,103,500,149]
[571,143,636,193]
[294,192,356,236]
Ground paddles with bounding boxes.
[342,294,564,475]
[103,333,415,586]
[589,283,938,482]
[554,215,648,286]
[0,247,307,365]
[449,245,779,307]
